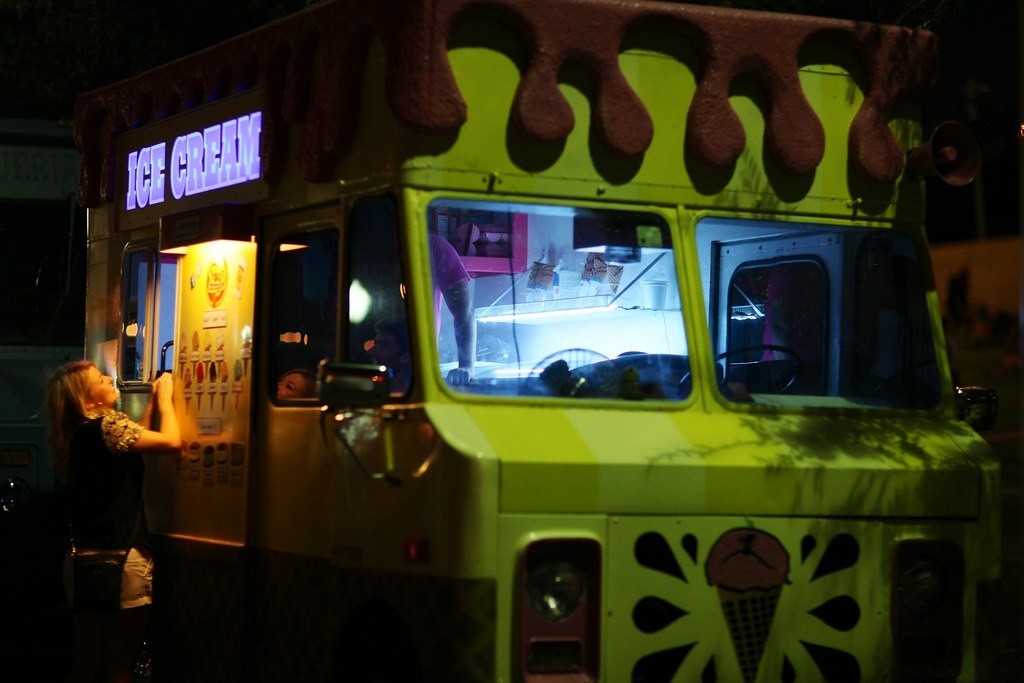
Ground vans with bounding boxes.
[1,116,82,516]
[79,2,1009,683]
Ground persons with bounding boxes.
[429,232,475,385]
[46,361,183,683]
[371,318,410,392]
[274,369,315,398]
